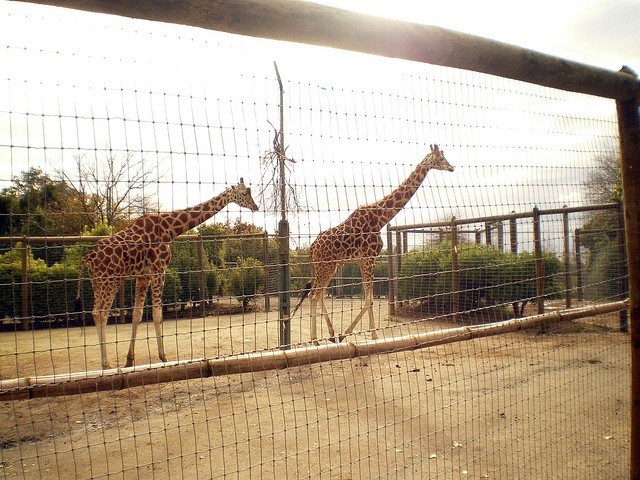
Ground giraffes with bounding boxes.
[73,178,259,371]
[290,144,454,343]
